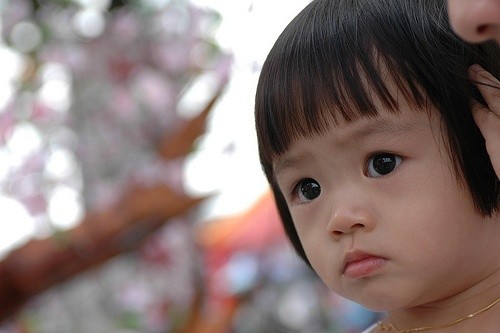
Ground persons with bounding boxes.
[252,1,500,333]
[450,0,500,43]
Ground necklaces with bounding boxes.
[375,293,500,333]
